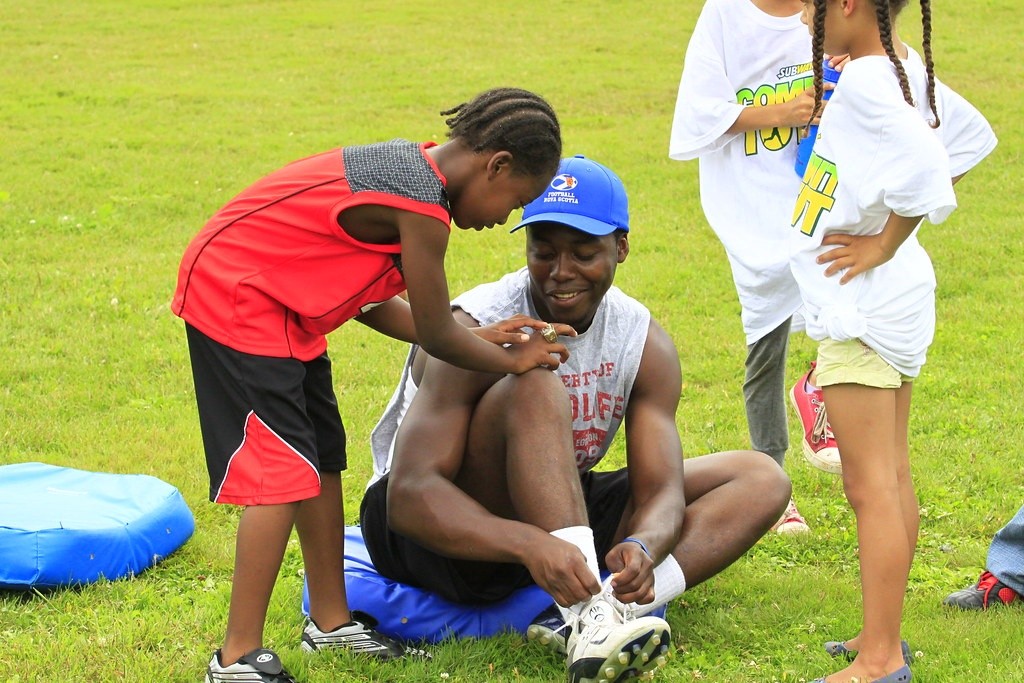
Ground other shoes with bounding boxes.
[819,634,913,665]
[802,665,913,683]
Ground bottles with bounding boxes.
[796,61,840,177]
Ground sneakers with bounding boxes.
[943,572,1018,608]
[299,612,435,664]
[551,593,671,682]
[769,496,807,535]
[203,646,298,683]
[527,568,671,658]
[790,361,842,474]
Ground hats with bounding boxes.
[509,152,631,238]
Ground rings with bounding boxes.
[540,323,558,344]
[359,150,791,683]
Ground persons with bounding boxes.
[170,87,582,683]
[788,2,998,683]
[668,1,929,543]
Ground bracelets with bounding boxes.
[621,537,652,562]
